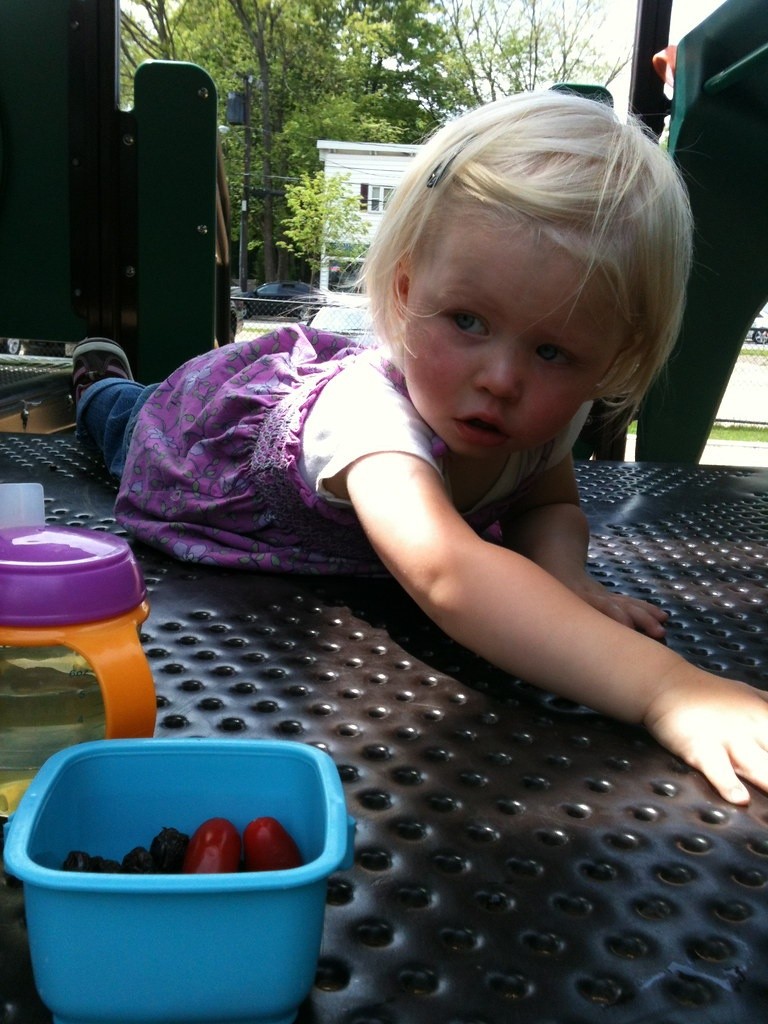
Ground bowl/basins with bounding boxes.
[2,738,356,1016]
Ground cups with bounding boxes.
[0,523,156,818]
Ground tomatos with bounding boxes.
[180,817,300,874]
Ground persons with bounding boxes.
[71,93,768,806]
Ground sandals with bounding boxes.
[73,337,135,408]
